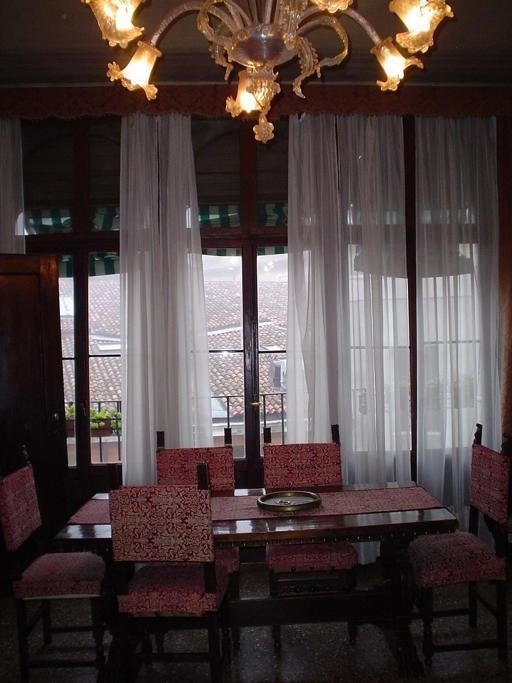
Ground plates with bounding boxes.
[258,490,323,511]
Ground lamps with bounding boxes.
[78,0,455,145]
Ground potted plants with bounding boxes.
[64,402,122,438]
